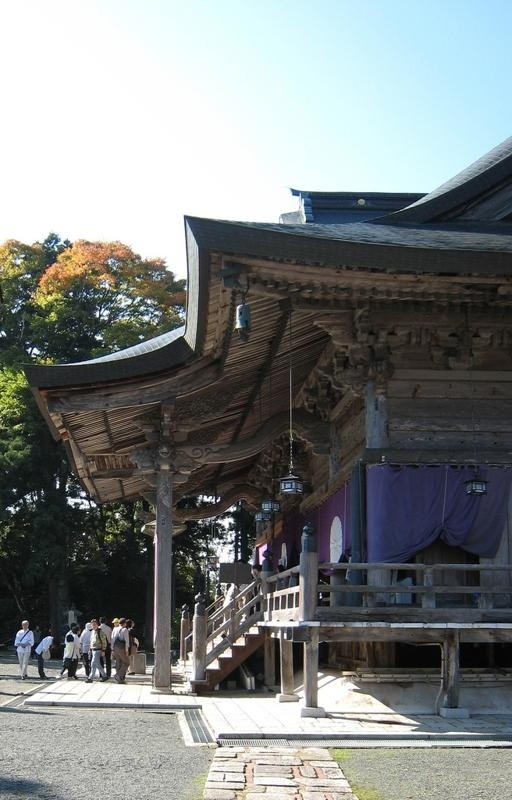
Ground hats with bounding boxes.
[112,618,119,623]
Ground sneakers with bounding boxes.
[85,676,126,683]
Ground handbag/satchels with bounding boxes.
[64,658,73,668]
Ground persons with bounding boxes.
[13,620,34,679]
[44,626,55,659]
[221,583,242,638]
[33,625,41,659]
[171,634,177,656]
[35,634,61,680]
[238,548,299,621]
[62,616,138,684]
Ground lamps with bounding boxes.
[462,301,489,497]
[254,298,305,522]
